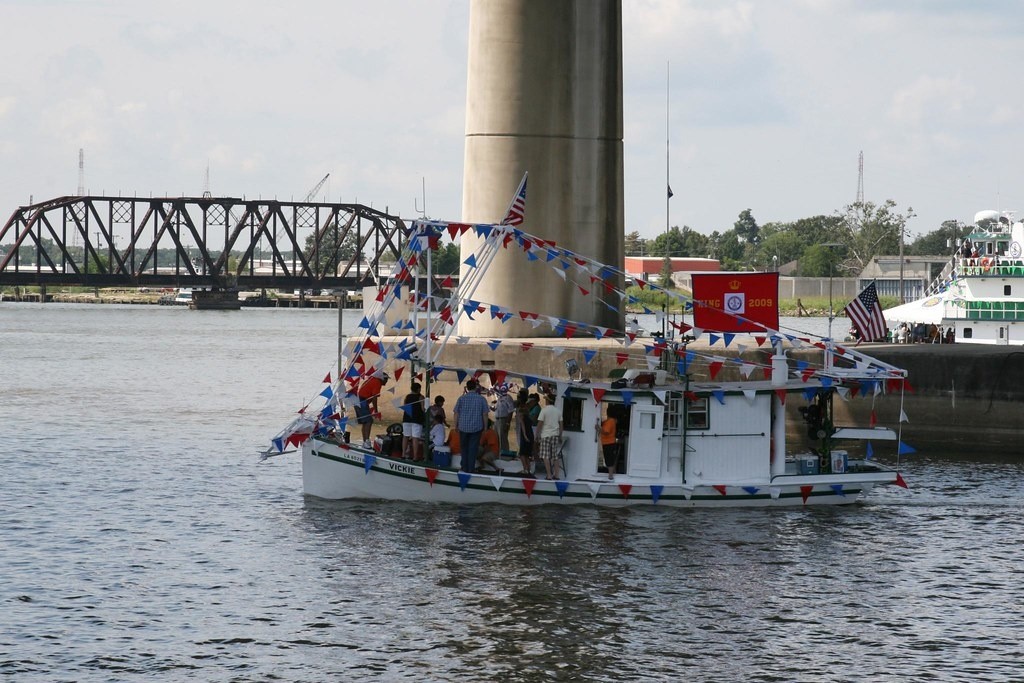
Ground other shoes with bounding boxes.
[362,439,372,449]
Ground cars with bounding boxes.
[176,292,193,302]
[139,286,165,293]
[158,296,175,302]
[294,289,306,296]
[320,289,332,296]
[330,289,347,297]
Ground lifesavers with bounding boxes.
[981,258,994,271]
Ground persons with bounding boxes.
[353,372,389,449]
[478,418,504,476]
[491,388,542,473]
[595,405,619,480]
[453,379,490,472]
[536,393,564,480]
[899,323,953,343]
[426,396,460,458]
[401,380,426,461]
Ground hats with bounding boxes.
[543,395,553,402]
[382,372,391,379]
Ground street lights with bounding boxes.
[947,220,957,280]
[899,219,907,305]
[946,237,961,280]
[818,243,844,339]
[94,232,100,255]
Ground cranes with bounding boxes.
[265,174,330,252]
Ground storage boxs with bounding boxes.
[431,446,451,467]
[800,455,819,476]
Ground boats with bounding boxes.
[302,172,907,508]
[882,233,1024,345]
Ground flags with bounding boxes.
[492,174,528,238]
[844,282,888,342]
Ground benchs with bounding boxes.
[450,454,535,474]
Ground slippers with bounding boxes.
[552,476,560,480]
[544,476,552,480]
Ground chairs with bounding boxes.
[547,438,568,478]
